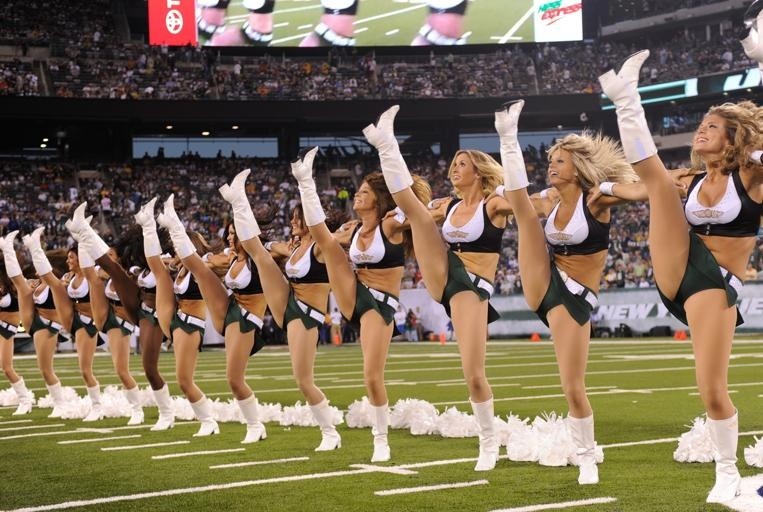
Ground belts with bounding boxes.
[420,24,465,45]
[242,21,274,42]
[197,16,229,34]
[314,22,355,47]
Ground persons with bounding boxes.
[318,308,356,345]
[0,193,231,437]
[493,99,691,486]
[286,146,450,464]
[0,0,763,295]
[216,167,360,450]
[402,306,422,342]
[363,102,560,473]
[261,310,281,345]
[597,46,763,505]
